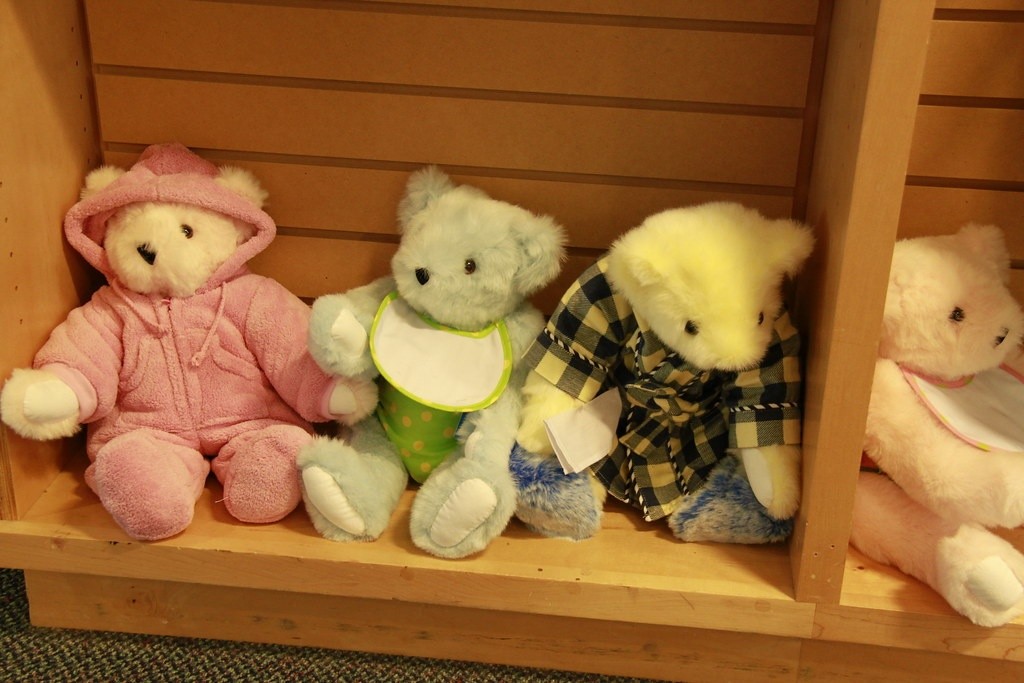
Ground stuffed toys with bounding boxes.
[846,221,1024,630]
[293,164,572,562]
[1,140,381,541]
[509,201,814,545]
